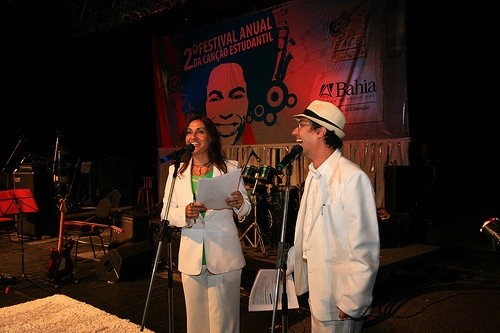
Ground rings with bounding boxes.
[235,200,238,204]
[190,203,193,208]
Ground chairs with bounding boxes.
[65,197,112,271]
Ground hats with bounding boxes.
[294,99,346,139]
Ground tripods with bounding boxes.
[0,188,42,290]
[239,194,270,257]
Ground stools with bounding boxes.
[136,176,157,215]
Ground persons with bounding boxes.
[160,114,252,333]
[280,100,380,333]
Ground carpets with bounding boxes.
[0,294,156,333]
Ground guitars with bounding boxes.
[45,198,75,279]
[63,220,124,234]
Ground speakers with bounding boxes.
[119,214,153,243]
[96,241,156,282]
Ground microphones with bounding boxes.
[277,145,303,172]
[248,147,261,162]
[159,144,196,165]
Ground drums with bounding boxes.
[256,164,275,184]
[240,163,258,183]
[268,204,284,227]
[270,185,300,210]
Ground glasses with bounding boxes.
[296,122,312,130]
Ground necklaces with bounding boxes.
[192,161,210,174]
